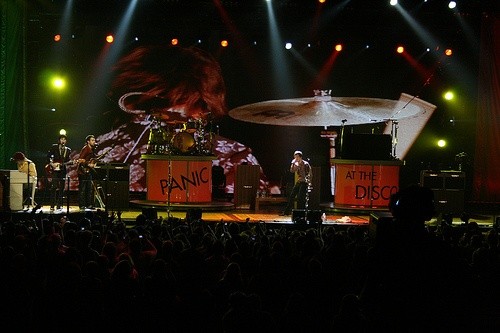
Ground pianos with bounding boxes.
[0,170,37,210]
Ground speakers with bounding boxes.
[186,209,202,220]
[143,207,157,219]
[291,210,323,223]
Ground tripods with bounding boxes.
[23,161,38,207]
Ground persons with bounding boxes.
[15,152,36,177]
[76,135,108,210]
[46,135,79,210]
[0,203,500,333]
[280,151,313,215]
[84,42,281,196]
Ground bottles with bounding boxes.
[322,212,327,223]
[256,189,272,199]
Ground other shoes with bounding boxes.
[57,205,62,210]
[80,206,86,209]
[50,206,54,210]
[88,205,94,209]
[279,212,288,216]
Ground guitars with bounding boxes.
[44,158,85,178]
[77,144,115,175]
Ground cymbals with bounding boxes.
[228,96,421,126]
[137,112,159,116]
[192,112,211,116]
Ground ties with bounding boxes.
[60,148,64,156]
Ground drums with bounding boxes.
[155,127,170,144]
[170,131,196,155]
[149,129,163,145]
[170,122,185,131]
[183,122,197,133]
